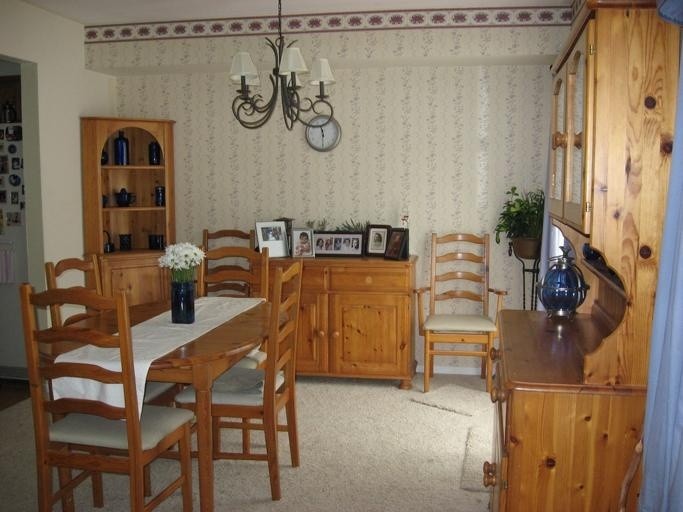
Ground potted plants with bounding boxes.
[494,187,545,259]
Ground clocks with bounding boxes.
[305,116,341,152]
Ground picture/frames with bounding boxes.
[255,220,409,262]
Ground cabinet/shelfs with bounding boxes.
[80,117,176,314]
[247,255,419,390]
[548,1,681,236]
[484,310,647,512]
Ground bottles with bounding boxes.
[114,131,129,165]
[148,136,160,165]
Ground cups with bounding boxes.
[155,186,165,206]
[119,234,132,250]
[149,234,163,250]
[116,194,137,207]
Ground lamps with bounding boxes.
[229,0,336,130]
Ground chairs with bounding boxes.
[414,233,507,393]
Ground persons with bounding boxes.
[343,238,349,251]
[334,238,341,250]
[316,239,325,252]
[260,227,282,240]
[325,239,331,251]
[389,232,403,257]
[352,239,359,250]
[296,232,311,255]
[373,233,383,250]
